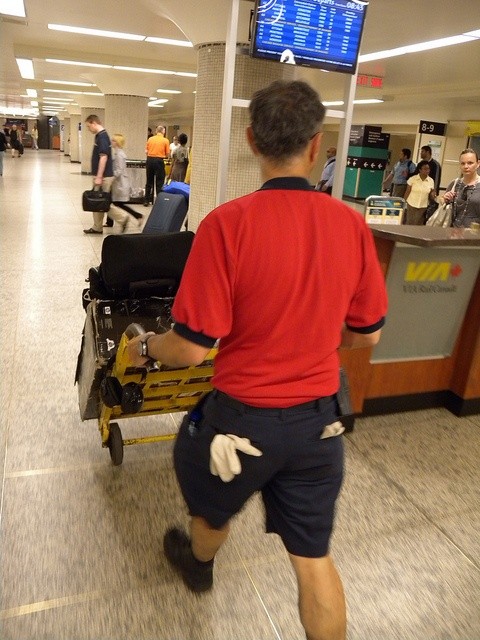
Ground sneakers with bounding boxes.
[163,528,215,592]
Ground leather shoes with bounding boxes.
[85,228,103,234]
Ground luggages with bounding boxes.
[141,191,187,236]
[89,267,179,311]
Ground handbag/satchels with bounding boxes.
[82,184,111,212]
[98,231,195,293]
[424,190,439,226]
[425,178,460,227]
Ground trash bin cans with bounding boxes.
[124,159,146,203]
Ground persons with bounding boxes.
[171,133,190,185]
[445,150,480,227]
[0,130,8,176]
[103,133,144,231]
[417,146,441,198]
[9,125,23,158]
[31,125,39,151]
[385,146,416,197]
[3,125,10,146]
[148,127,153,139]
[404,162,443,225]
[125,79,388,638]
[315,145,336,195]
[169,135,180,158]
[84,114,129,234]
[144,125,171,206]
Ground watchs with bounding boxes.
[133,332,153,361]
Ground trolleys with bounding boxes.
[362,194,406,227]
[97,322,218,466]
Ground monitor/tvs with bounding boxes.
[249,0,368,75]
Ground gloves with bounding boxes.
[209,432,264,482]
